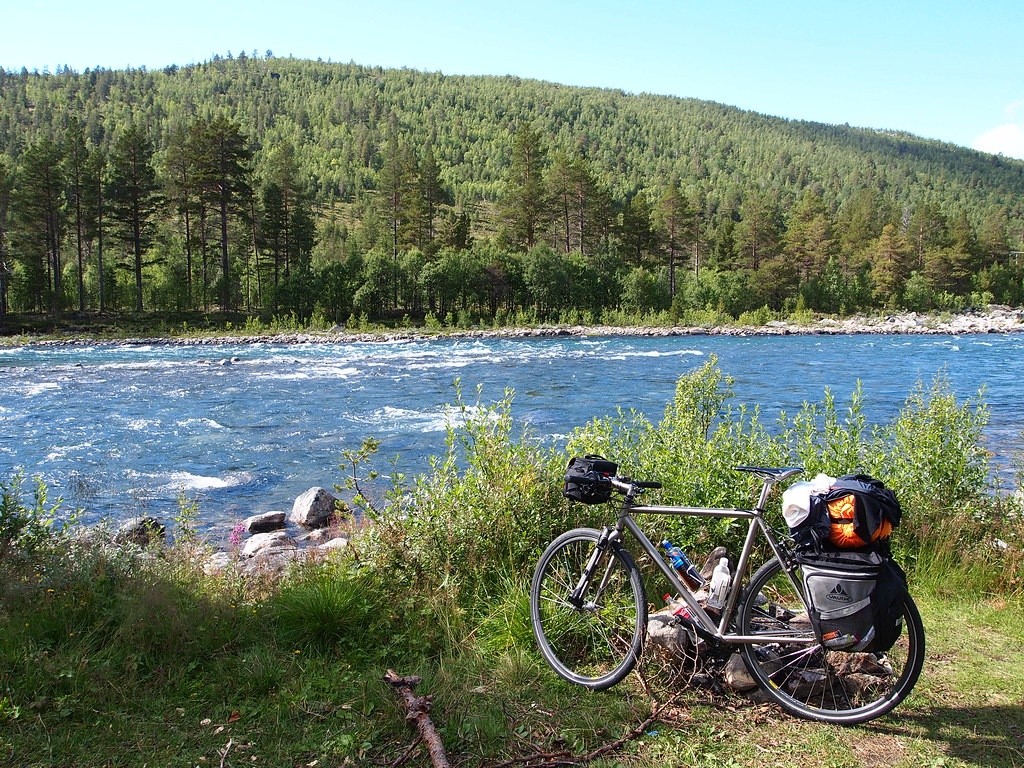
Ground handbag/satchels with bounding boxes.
[562,455,617,504]
[795,550,906,652]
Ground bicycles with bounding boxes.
[523,465,925,725]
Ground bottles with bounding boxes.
[707,557,730,609]
[661,593,706,645]
[662,541,706,593]
